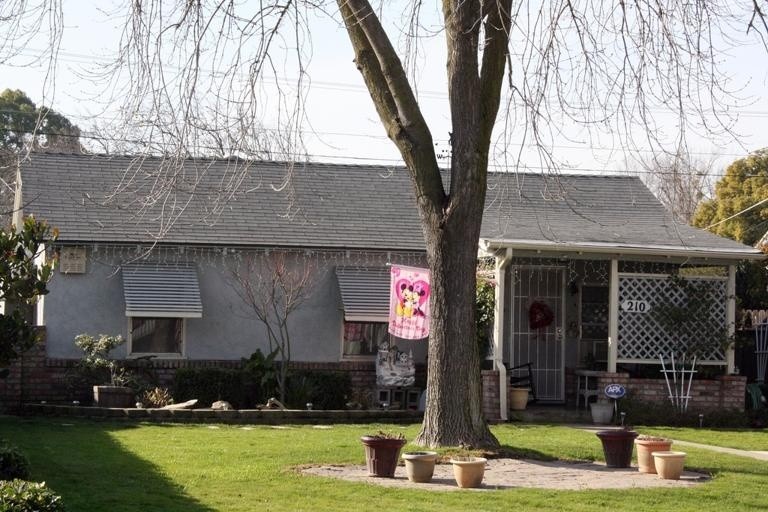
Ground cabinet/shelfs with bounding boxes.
[577,280,611,368]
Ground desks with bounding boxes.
[575,367,607,409]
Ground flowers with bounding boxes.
[528,300,553,332]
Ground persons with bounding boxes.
[376,341,412,373]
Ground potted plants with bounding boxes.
[90,358,145,410]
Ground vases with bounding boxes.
[362,435,407,478]
[508,385,531,410]
[634,438,673,476]
[450,451,488,488]
[404,450,439,485]
[589,402,614,424]
[595,425,639,470]
[649,450,687,480]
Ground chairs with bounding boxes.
[504,359,539,403]
